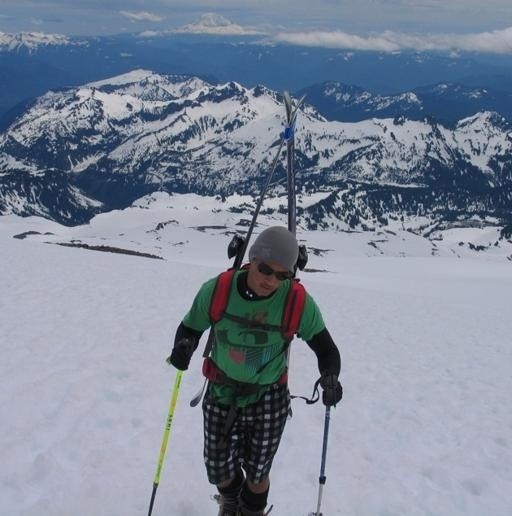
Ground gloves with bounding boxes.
[320,372,342,406]
[171,338,192,370]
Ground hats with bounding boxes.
[248,226,299,274]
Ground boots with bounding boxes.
[216,472,269,516]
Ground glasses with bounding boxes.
[254,256,290,280]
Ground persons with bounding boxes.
[170,226,342,516]
